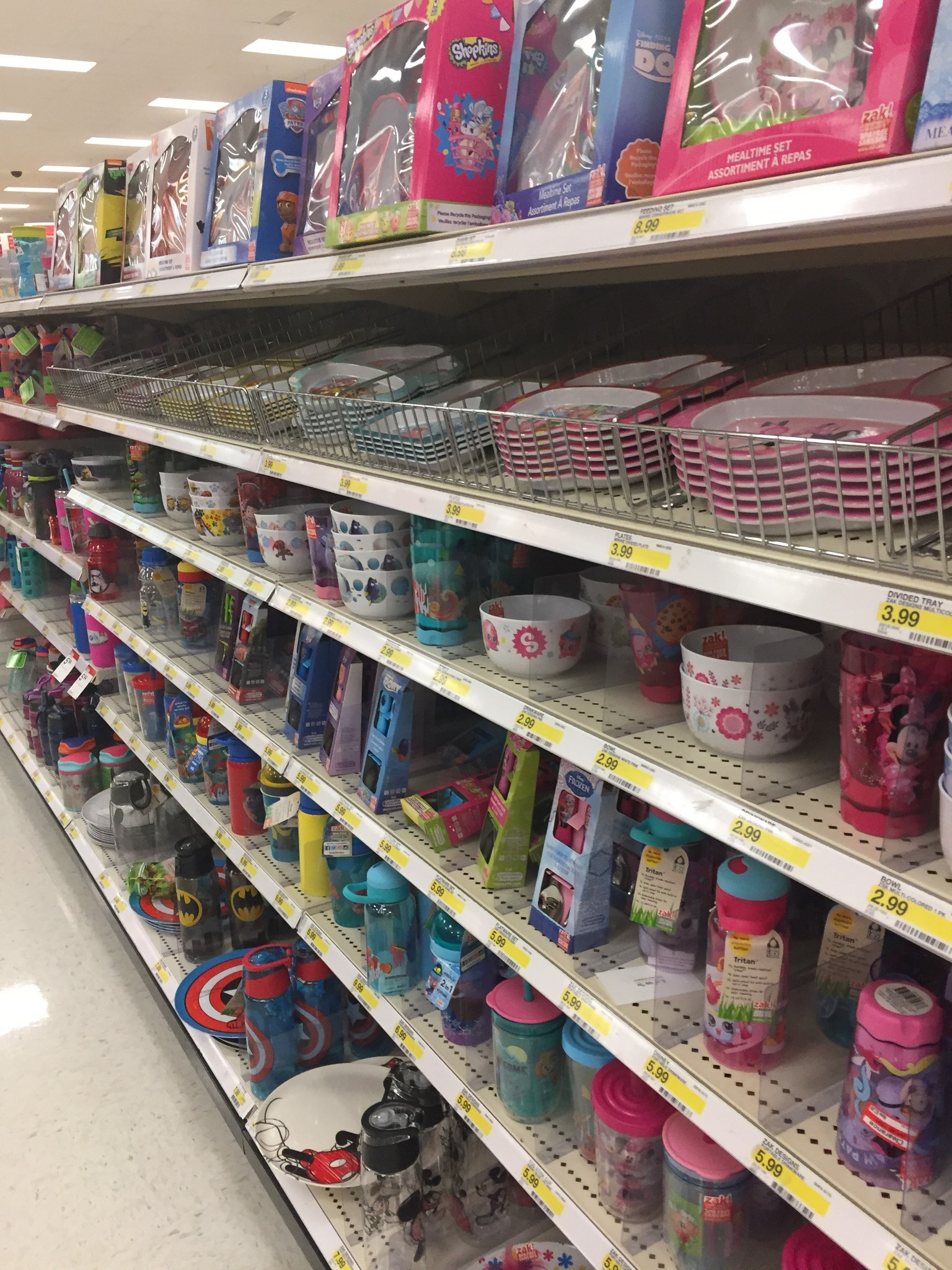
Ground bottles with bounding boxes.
[0,223,51,299]
[1,443,952,1269]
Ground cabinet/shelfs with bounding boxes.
[244,148,952,1270]
[54,147,254,1120]
[0,294,79,828]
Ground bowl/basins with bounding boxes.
[68,439,951,880]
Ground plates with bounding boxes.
[251,1063,450,1190]
[63,327,951,536]
[470,1242,589,1269]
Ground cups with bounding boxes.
[617,577,699,703]
[127,439,163,514]
[234,470,286,563]
[475,528,533,604]
[412,511,467,646]
[303,505,340,603]
[841,628,951,836]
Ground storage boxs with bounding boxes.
[52,184,77,292]
[491,0,688,224]
[213,584,243,678]
[286,624,338,749]
[227,596,269,704]
[120,146,151,284]
[324,0,513,248]
[199,80,308,268]
[477,734,539,888]
[527,760,622,956]
[652,0,941,197]
[145,112,217,278]
[292,63,344,256]
[357,670,415,813]
[319,645,363,775]
[401,765,559,851]
[74,159,125,288]
